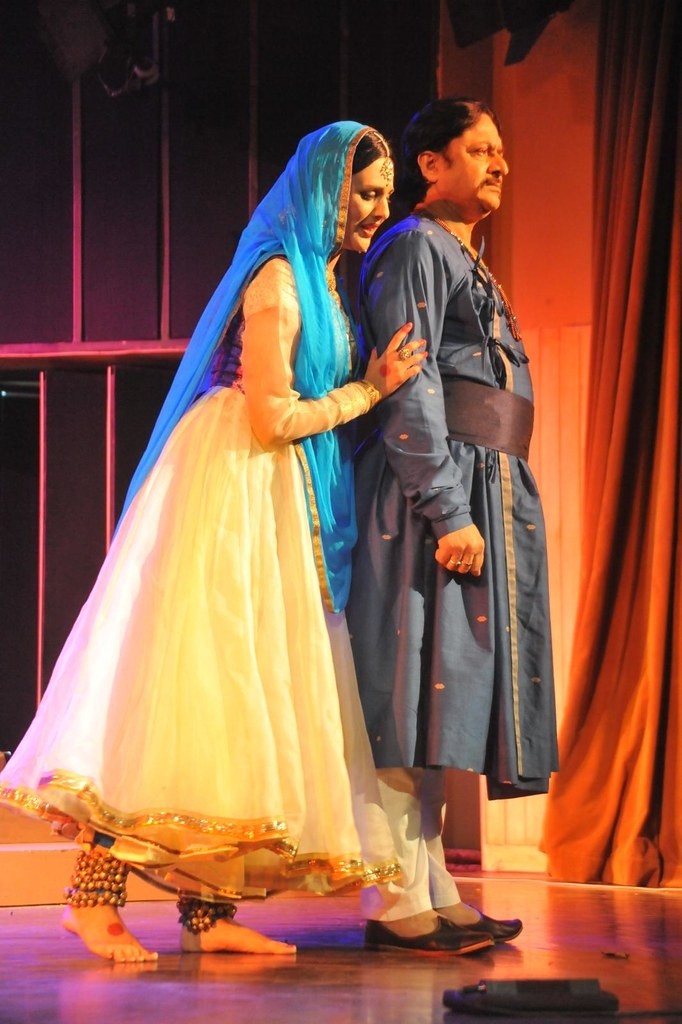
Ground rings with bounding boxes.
[399,349,410,360]
[449,560,462,566]
[462,561,473,566]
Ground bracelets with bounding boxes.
[357,380,376,408]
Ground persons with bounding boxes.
[0,116,428,963]
[346,94,561,959]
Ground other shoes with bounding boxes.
[364,918,495,957]
[437,904,523,942]
